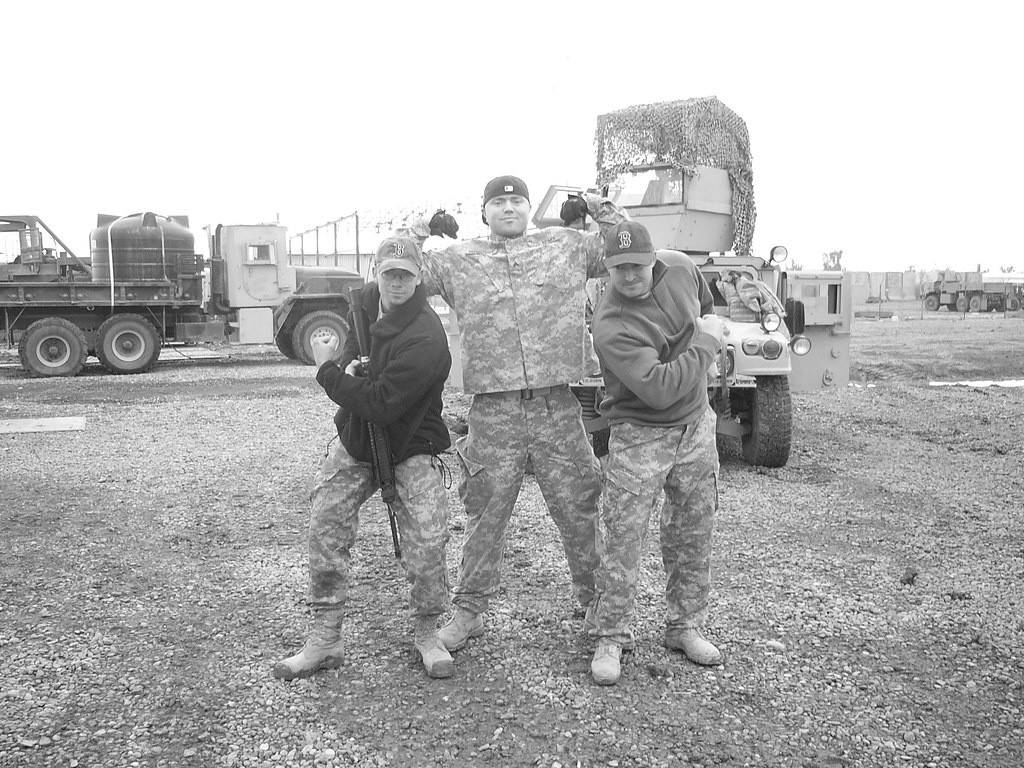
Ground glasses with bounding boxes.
[382,270,414,282]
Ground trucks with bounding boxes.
[924,264,1020,311]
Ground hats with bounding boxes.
[482,176,529,225]
[604,219,653,268]
[374,237,422,276]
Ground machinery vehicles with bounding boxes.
[0,212,367,378]
[531,97,810,468]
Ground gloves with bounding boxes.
[560,198,587,225]
[428,211,459,239]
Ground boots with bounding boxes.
[274,610,345,680]
[413,615,455,677]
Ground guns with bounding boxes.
[348,288,403,559]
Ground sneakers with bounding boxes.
[591,644,622,685]
[438,607,484,651]
[584,604,636,650]
[665,627,722,665]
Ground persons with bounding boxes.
[588,220,731,684]
[395,175,635,651]
[273,237,454,678]
